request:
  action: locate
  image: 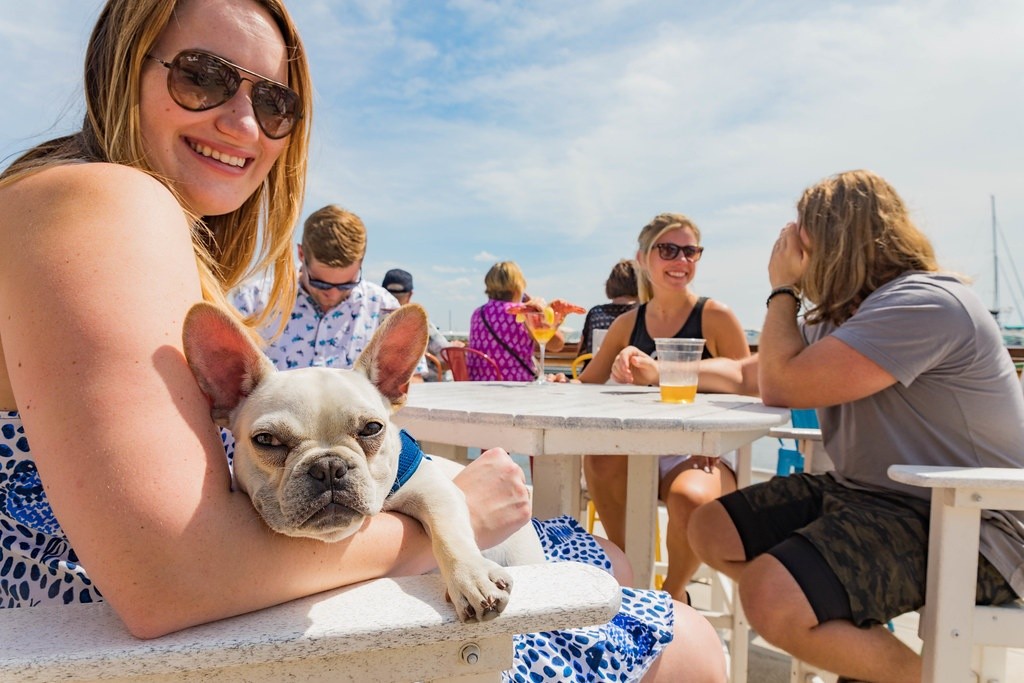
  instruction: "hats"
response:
[382,269,413,293]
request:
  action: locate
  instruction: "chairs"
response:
[0,561,622,683]
[424,350,442,381]
[440,347,534,482]
[578,327,733,647]
[572,354,663,594]
[767,422,1023,683]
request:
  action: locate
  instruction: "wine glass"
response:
[525,312,567,385]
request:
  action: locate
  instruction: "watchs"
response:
[766,286,802,316]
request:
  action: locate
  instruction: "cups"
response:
[653,337,707,404]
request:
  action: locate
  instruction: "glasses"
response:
[652,243,703,263]
[305,257,362,289]
[145,49,303,140]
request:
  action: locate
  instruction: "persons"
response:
[382,268,465,382]
[471,261,566,381]
[542,212,750,613]
[224,204,431,387]
[0,0,729,683]
[609,169,1023,683]
[578,262,642,357]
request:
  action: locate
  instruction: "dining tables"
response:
[390,380,792,683]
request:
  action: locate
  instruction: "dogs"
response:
[182,300,548,622]
